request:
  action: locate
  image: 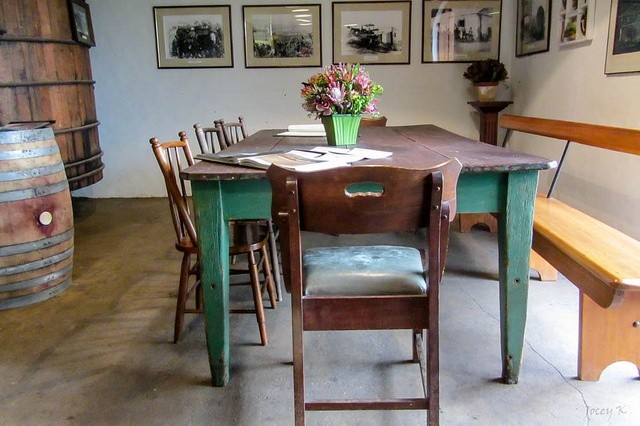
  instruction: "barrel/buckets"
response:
[0,129,74,311]
[0,1,105,190]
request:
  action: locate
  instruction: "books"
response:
[309,146,393,162]
[274,124,327,137]
[193,150,351,172]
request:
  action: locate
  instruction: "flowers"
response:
[299,61,384,119]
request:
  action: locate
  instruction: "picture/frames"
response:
[421,0,502,64]
[67,0,96,47]
[557,0,595,45]
[152,4,234,69]
[515,0,552,58]
[332,1,412,65]
[603,0,640,75]
[243,4,322,69]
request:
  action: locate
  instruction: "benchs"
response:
[458,113,640,381]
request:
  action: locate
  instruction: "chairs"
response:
[221,116,248,147]
[193,120,283,301]
[150,132,277,346]
[265,157,467,426]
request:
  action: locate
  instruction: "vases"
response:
[321,114,361,147]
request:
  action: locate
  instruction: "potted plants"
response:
[462,58,508,102]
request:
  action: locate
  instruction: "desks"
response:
[181,124,557,388]
